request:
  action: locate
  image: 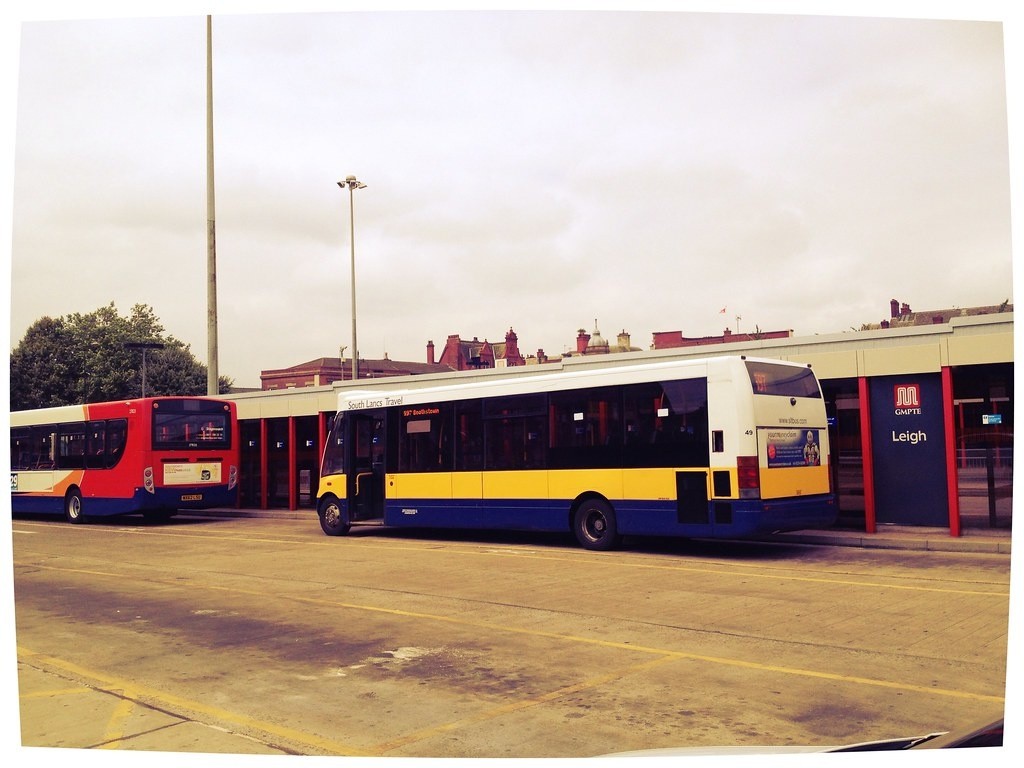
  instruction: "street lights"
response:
[336,175,368,381]
[124,342,166,399]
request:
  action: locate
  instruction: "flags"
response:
[719,308,725,313]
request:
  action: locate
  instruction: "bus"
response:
[316,354,838,551]
[10,394,241,522]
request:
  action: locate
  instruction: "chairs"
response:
[36,447,121,470]
[515,454,548,468]
[417,452,447,472]
